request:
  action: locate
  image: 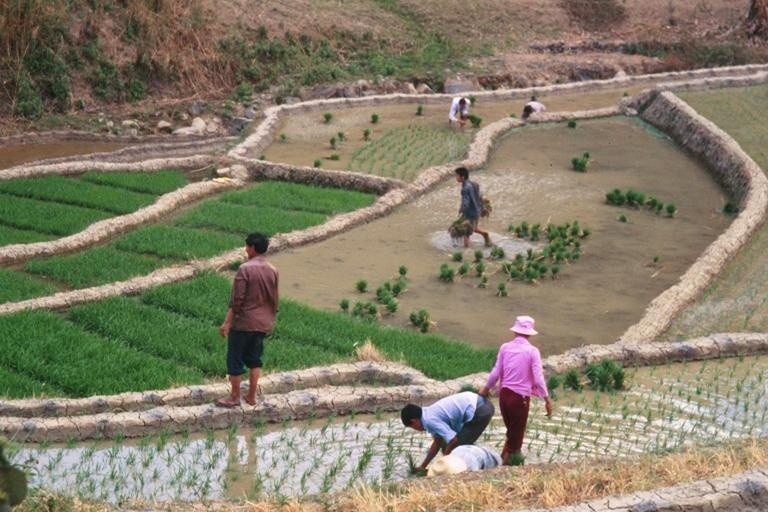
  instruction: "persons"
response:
[217,232,279,406]
[454,167,491,249]
[428,443,504,475]
[521,100,547,117]
[447,96,471,127]
[478,315,552,465]
[401,391,494,471]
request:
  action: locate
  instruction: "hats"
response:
[508,314,539,335]
